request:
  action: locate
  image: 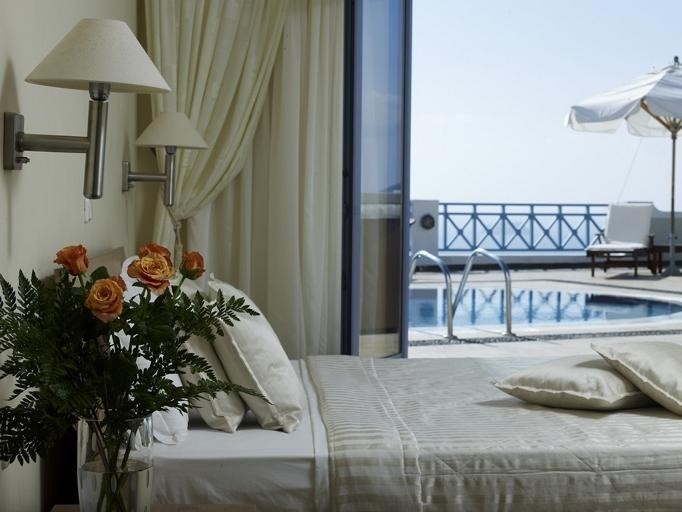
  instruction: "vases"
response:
[73,413,153,507]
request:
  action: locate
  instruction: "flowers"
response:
[1,239,266,510]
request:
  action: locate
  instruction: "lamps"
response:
[120,111,210,207]
[3,17,168,198]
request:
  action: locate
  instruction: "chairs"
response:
[584,201,662,278]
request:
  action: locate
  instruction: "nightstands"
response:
[48,502,268,511]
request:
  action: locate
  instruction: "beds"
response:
[72,352,682,511]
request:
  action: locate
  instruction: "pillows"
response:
[501,353,648,411]
[105,253,189,442]
[152,271,250,432]
[208,274,304,434]
[595,343,682,416]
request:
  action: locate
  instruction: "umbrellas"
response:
[568,54,681,280]
